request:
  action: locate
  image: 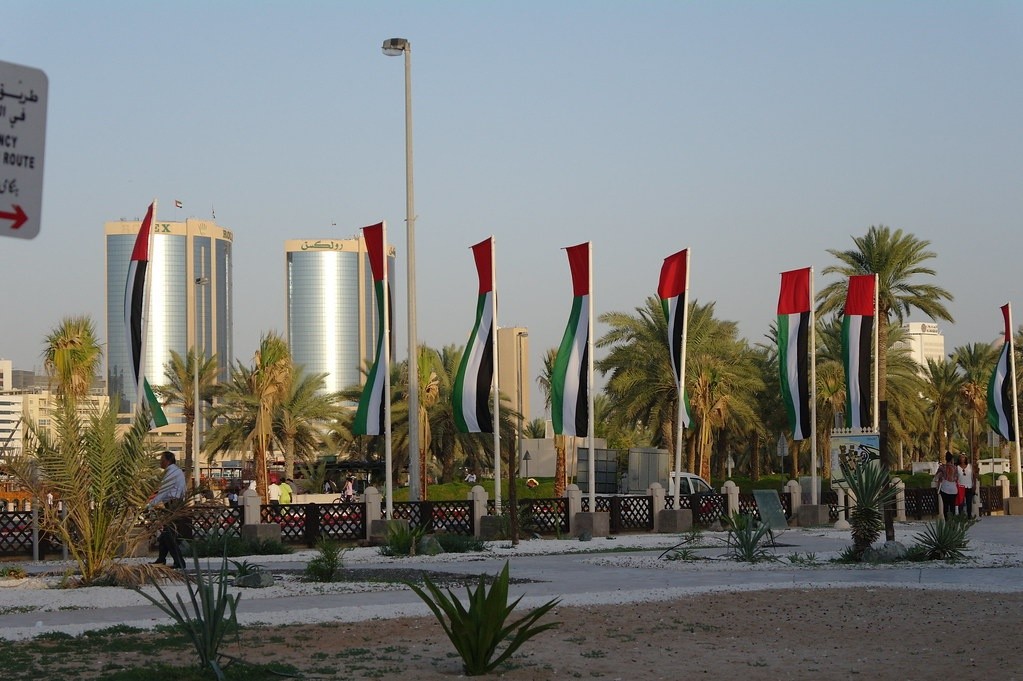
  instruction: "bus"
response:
[198,453,436,489]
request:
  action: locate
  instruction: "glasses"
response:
[962,469,967,476]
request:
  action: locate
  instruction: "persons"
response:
[322,477,355,503]
[143,451,187,569]
[228,479,293,517]
[934,451,977,521]
[464,470,476,481]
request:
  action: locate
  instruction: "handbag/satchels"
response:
[955,484,965,506]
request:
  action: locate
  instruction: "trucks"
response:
[668,472,717,512]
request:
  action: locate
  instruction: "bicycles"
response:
[332,494,362,513]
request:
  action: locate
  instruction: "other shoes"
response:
[150,559,167,564]
[171,562,186,568]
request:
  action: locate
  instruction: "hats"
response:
[271,478,277,482]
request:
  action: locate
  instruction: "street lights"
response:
[518,333,529,474]
[194,276,209,507]
[380,37,419,554]
